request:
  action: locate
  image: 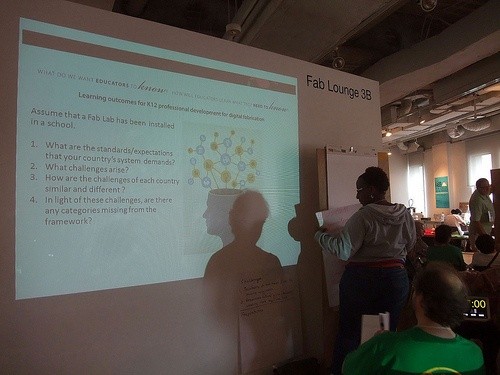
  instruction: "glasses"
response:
[356,185,369,193]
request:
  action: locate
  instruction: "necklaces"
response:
[373,199,387,205]
[417,323,452,330]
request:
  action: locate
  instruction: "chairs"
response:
[462,269,498,328]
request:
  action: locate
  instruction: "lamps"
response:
[328,47,345,70]
[226,0,241,36]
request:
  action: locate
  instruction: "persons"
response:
[313,166,500,375]
[201,188,243,250]
